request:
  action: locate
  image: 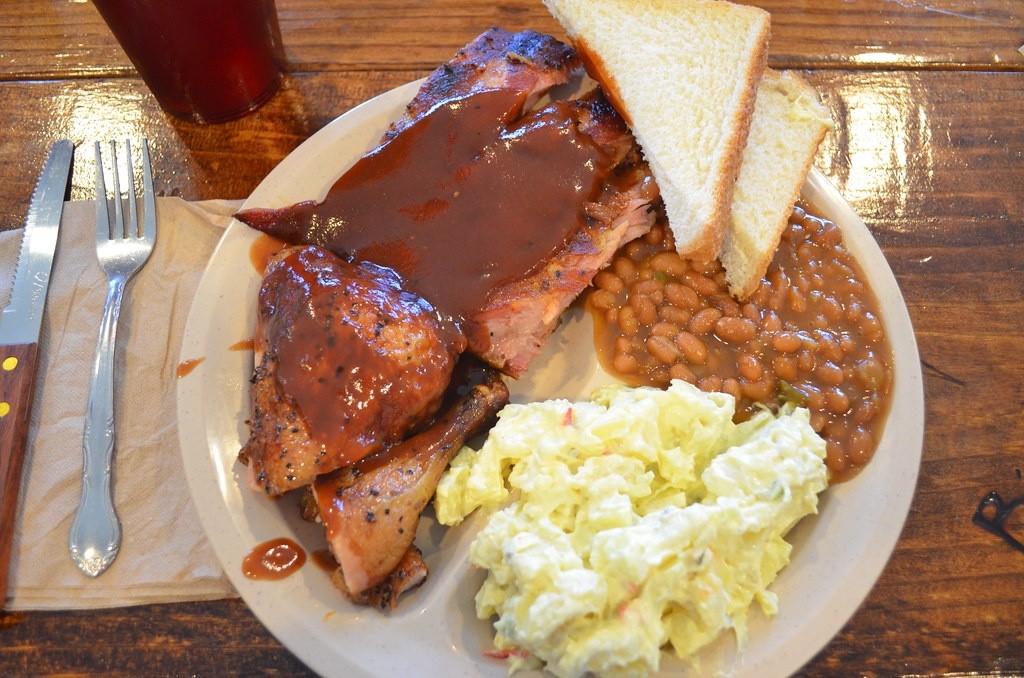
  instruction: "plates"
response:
[177,75,924,678]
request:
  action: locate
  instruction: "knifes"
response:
[0,139,75,612]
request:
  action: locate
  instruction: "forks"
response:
[66,141,158,578]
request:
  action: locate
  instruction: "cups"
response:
[92,0,288,125]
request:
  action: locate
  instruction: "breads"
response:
[542,0,772,262]
[718,66,836,302]
